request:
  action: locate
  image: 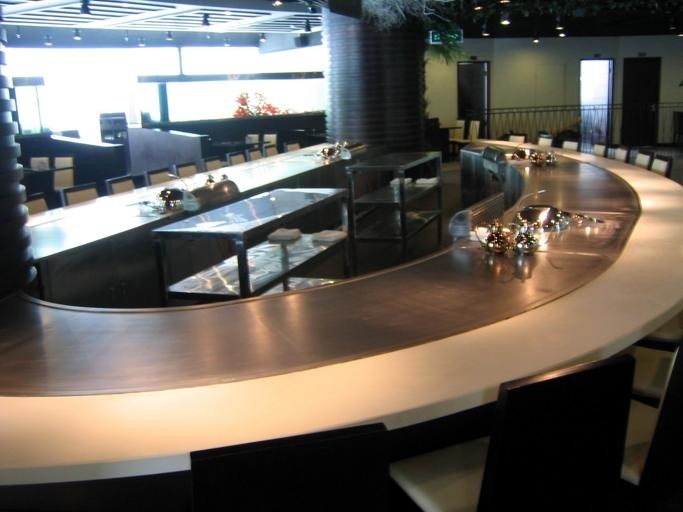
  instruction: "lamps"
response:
[13,3,569,48]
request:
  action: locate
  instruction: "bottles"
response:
[530,151,559,165]
[473,222,538,256]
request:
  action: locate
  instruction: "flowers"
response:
[231,92,289,120]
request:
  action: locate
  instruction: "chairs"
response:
[0,111,682,511]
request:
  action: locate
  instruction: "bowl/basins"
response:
[321,147,341,158]
[159,187,183,208]
